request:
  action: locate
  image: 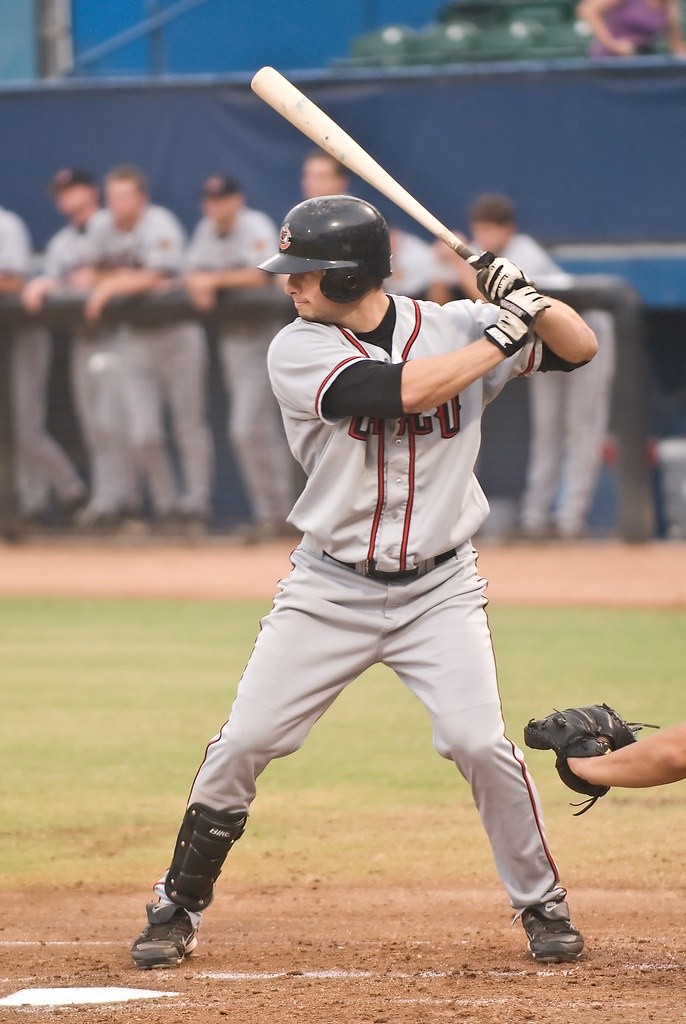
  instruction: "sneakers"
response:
[130,902,198,969]
[522,900,584,963]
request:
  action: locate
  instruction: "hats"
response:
[49,169,86,193]
[203,176,238,198]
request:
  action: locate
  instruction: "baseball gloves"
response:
[523,702,661,817]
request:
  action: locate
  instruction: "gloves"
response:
[474,250,527,306]
[484,279,552,358]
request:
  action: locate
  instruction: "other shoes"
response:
[0,490,302,545]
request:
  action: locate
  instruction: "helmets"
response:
[257,195,393,304]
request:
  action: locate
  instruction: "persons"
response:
[576,0,686,60]
[523,703,685,816]
[0,148,617,545]
[130,196,598,968]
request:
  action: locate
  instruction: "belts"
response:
[323,548,456,581]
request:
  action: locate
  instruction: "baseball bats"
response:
[251,65,481,271]
